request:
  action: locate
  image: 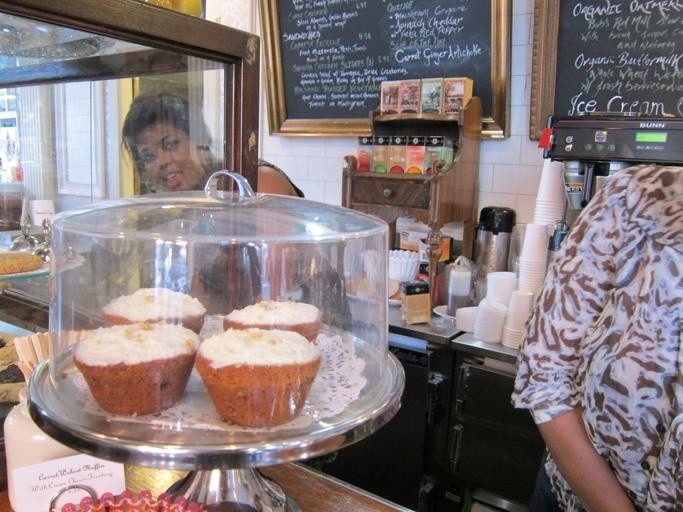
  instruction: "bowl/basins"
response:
[361,250,420,282]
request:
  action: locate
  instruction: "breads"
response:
[0,252,43,274]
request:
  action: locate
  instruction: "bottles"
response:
[447,256,471,316]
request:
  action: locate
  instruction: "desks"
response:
[1,316,417,512]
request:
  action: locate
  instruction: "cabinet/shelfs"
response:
[299,326,451,512]
[450,339,547,512]
[341,97,483,316]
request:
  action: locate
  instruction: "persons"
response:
[116,87,354,473]
[510,161,682,512]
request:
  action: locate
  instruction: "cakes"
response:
[195,325,321,429]
[72,320,201,417]
[102,287,208,335]
[222,297,322,343]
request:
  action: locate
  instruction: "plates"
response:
[433,305,455,321]
[352,277,400,300]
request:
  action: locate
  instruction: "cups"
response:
[475,153,567,350]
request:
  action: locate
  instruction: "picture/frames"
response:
[55,79,107,203]
